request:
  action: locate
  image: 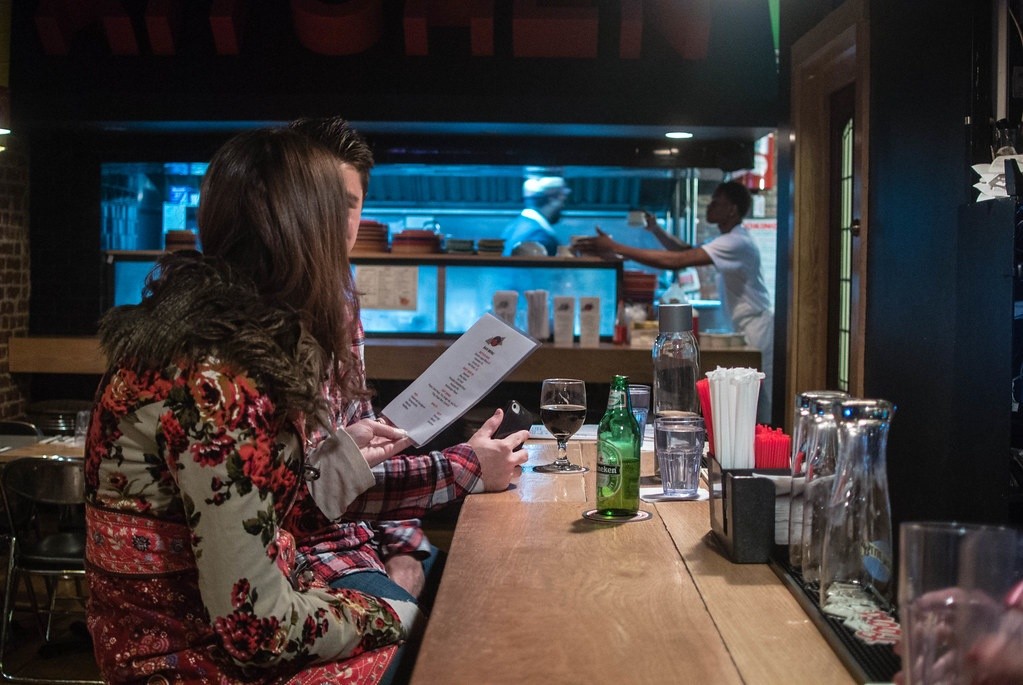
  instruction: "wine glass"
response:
[533,378,590,474]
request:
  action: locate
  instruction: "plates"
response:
[349,221,387,255]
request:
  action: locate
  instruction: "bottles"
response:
[653,305,699,480]
[597,375,640,517]
[612,278,721,348]
[790,390,893,619]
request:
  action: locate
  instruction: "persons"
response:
[571,180,775,419]
[500,177,573,260]
[85,114,532,685]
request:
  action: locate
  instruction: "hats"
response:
[522,177,572,197]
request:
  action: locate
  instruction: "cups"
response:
[556,235,619,258]
[654,417,704,497]
[899,522,1016,685]
[627,211,649,227]
[629,385,651,448]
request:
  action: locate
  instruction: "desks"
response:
[409,433,859,685]
[0,438,90,472]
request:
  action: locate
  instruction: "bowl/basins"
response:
[165,230,196,251]
[477,240,503,255]
[448,240,473,254]
[391,230,439,254]
[512,240,548,256]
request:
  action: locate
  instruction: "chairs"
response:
[0,418,105,685]
